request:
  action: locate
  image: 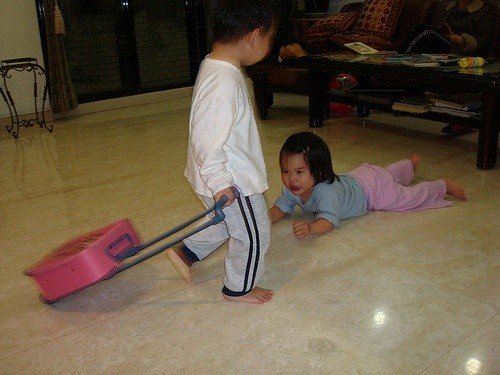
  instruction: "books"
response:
[344,42,448,68]
[392,87,484,118]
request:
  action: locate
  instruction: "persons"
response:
[166,0,275,304]
[268,131,467,239]
[397,0,495,55]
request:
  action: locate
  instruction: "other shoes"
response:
[441,122,477,135]
[371,73,410,86]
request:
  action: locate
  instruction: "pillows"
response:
[303,11,358,40]
[328,30,393,52]
[358,0,403,40]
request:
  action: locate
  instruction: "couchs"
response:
[247,12,310,121]
[335,0,500,118]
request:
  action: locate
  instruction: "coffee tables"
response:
[296,52,500,170]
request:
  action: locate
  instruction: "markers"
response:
[431,56,452,58]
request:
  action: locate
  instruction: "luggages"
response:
[24,187,239,305]
